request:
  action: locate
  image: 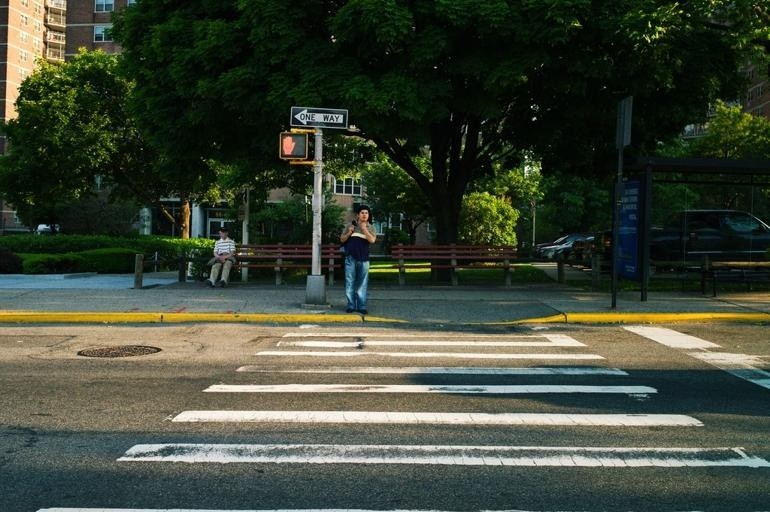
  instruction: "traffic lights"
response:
[278,127,310,157]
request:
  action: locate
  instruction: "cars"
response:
[648,207,769,274]
[533,230,615,263]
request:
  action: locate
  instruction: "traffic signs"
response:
[290,105,349,130]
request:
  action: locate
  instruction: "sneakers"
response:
[346,308,367,314]
[207,279,226,288]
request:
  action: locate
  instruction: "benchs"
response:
[391,242,519,287]
[228,242,342,287]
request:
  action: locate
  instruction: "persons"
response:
[207,227,237,287]
[340,205,377,314]
